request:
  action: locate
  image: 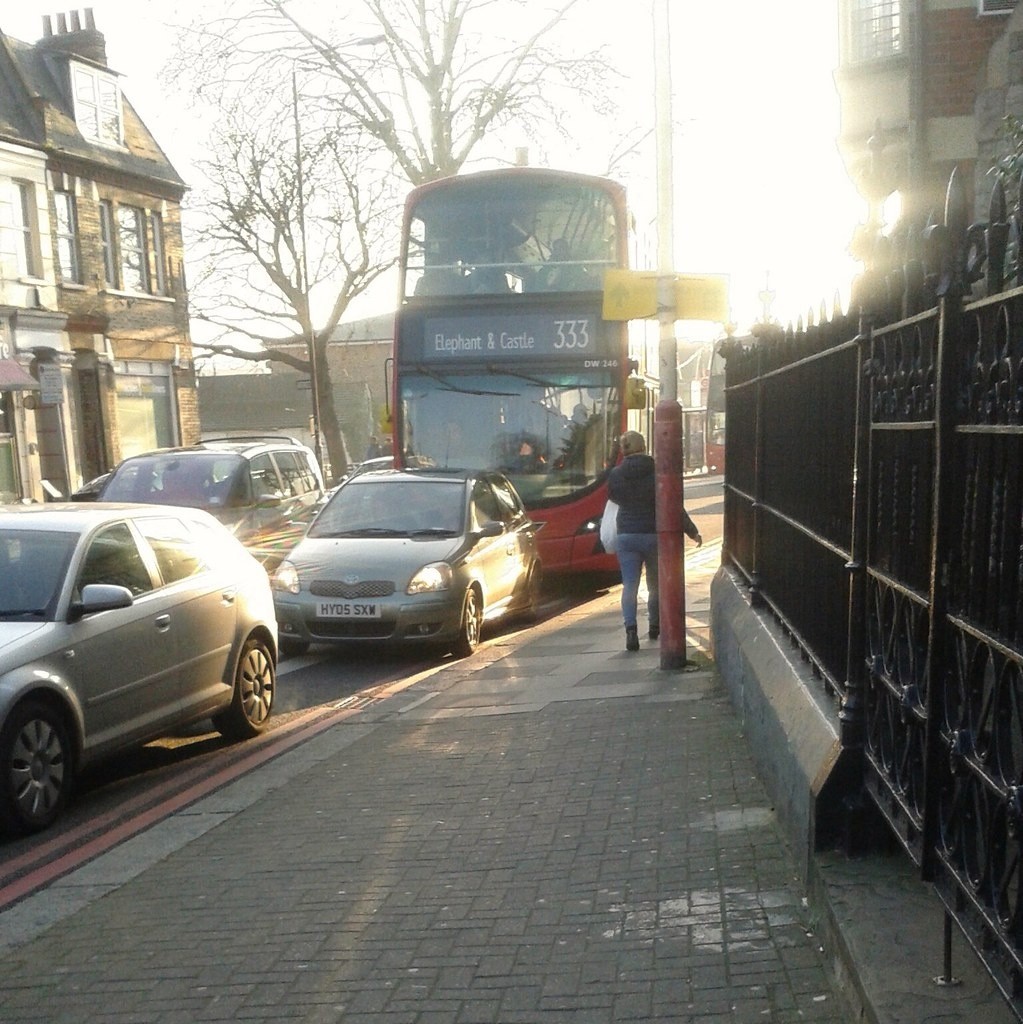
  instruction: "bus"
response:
[704,335,751,476]
[378,166,649,582]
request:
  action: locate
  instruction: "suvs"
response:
[97,436,325,561]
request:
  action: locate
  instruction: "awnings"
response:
[0,358,41,390]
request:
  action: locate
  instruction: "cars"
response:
[0,503,276,835]
[268,468,544,661]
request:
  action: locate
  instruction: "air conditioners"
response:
[977,0,1020,19]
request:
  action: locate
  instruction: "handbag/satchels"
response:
[600,499,620,554]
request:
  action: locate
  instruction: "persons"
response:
[571,402,590,422]
[539,239,599,291]
[365,436,393,460]
[607,430,702,652]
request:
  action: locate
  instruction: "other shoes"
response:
[649,625,659,639]
[627,630,639,651]
